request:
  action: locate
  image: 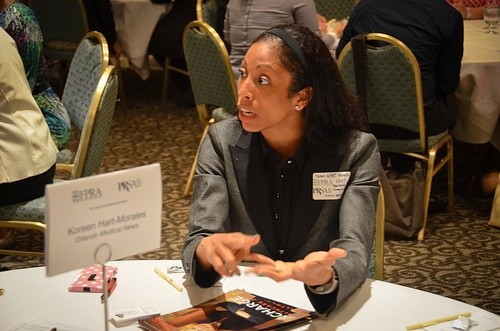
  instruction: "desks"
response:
[0,260,500,331]
[110,0,173,68]
[330,17,500,150]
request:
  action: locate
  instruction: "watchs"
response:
[308,270,334,293]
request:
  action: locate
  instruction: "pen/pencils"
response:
[155,267,183,292]
[408,313,474,330]
[101,277,116,302]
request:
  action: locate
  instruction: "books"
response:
[137,289,319,331]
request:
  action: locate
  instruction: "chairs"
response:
[0,0,453,279]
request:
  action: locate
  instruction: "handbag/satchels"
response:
[379,160,426,239]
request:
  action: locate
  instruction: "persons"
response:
[0,0,73,151]
[181,23,381,318]
[336,0,464,215]
[149,0,208,91]
[0,26,59,207]
[223,0,349,80]
